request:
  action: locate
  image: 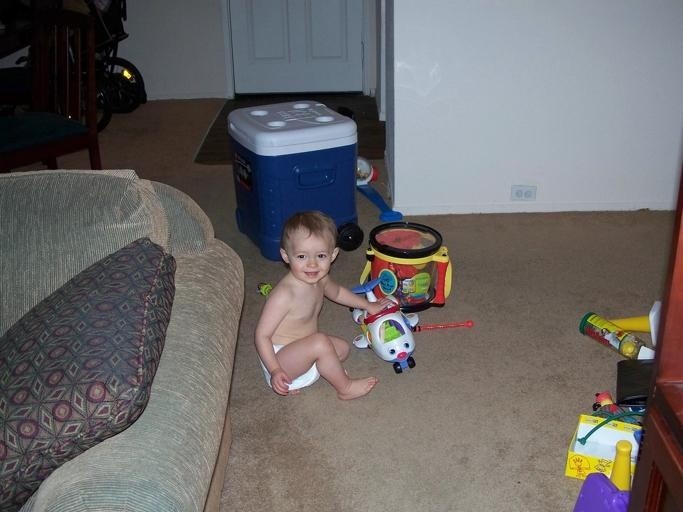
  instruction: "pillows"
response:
[0,237,176,512]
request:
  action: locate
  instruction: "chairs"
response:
[1,0,102,178]
[0,163,246,511]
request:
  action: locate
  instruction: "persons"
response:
[253,210,392,402]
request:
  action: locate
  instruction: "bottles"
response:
[578,311,647,361]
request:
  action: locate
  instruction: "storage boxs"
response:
[224,98,364,262]
[563,413,643,493]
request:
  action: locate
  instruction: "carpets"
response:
[192,88,385,166]
